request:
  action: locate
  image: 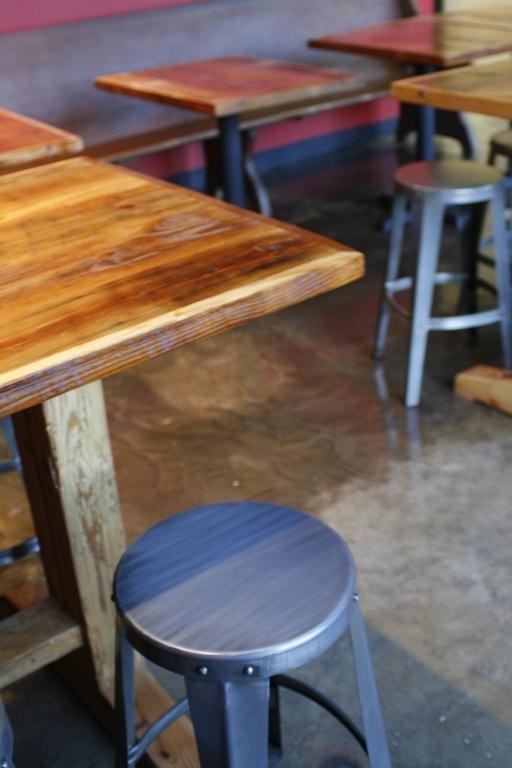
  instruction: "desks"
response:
[96,53,369,227]
[308,7,512,167]
[0,155,367,766]
[0,107,81,181]
[390,55,512,416]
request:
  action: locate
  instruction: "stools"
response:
[367,159,512,410]
[459,128,511,321]
[107,497,394,767]
